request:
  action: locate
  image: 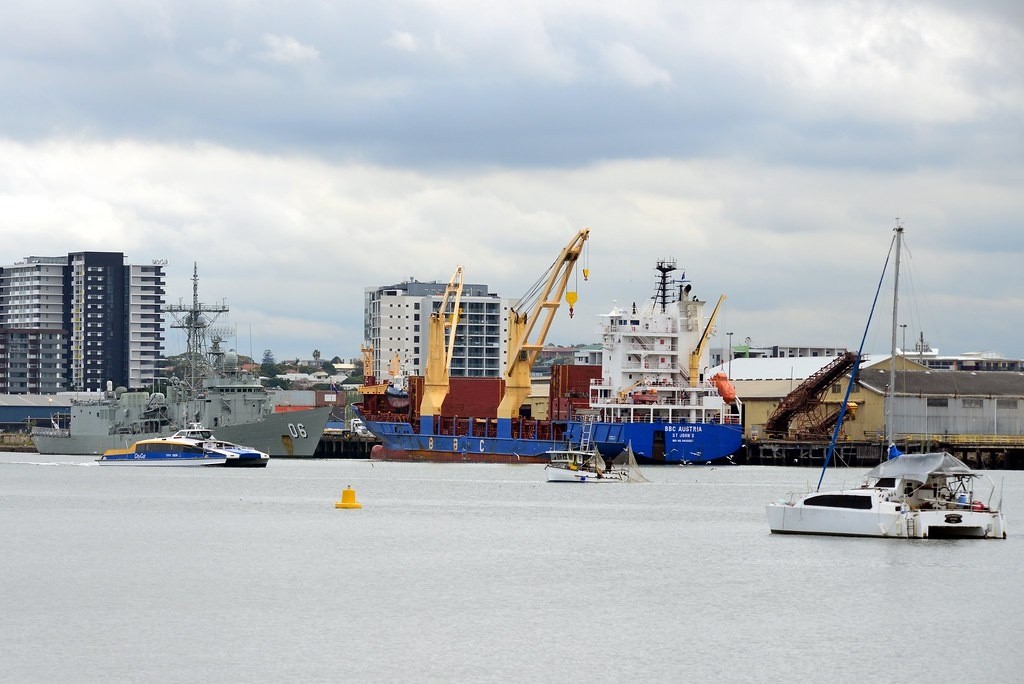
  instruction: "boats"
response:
[95,423,270,468]
[545,415,652,484]
[350,228,743,463]
[30,262,334,457]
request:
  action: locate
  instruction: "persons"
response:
[604,457,616,474]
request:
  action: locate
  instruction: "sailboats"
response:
[765,217,1007,538]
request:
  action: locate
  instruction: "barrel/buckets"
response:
[959,494,967,503]
